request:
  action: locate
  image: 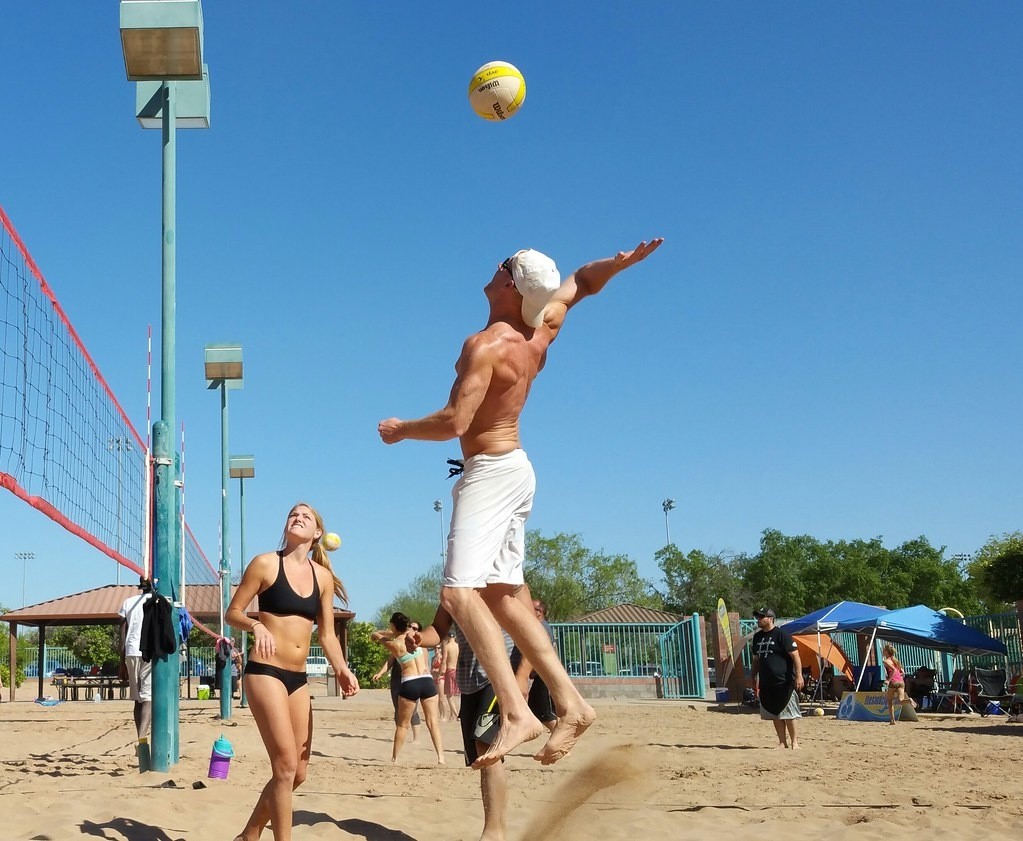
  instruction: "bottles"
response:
[137,737,150,774]
[95,693,100,703]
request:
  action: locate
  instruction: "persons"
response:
[905,666,933,696]
[230,637,243,700]
[401,573,533,841]
[375,237,664,773]
[749,608,805,750]
[370,611,451,766]
[225,501,361,841]
[944,669,974,712]
[117,574,152,755]
[433,632,462,720]
[882,644,917,725]
[530,598,561,733]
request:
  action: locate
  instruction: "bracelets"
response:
[251,622,262,630]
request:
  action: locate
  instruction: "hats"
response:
[138,576,150,589]
[513,248,561,329]
[752,607,776,621]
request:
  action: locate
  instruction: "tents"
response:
[834,605,1011,695]
[779,601,890,680]
[723,622,857,691]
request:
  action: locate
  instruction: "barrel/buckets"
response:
[989,700,1000,714]
[715,687,730,702]
[208,733,235,779]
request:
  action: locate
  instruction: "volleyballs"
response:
[322,533,341,553]
[813,707,825,716]
[468,60,527,121]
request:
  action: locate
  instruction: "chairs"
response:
[799,664,1017,718]
[200,675,216,698]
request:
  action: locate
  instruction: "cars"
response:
[305,656,329,677]
[23,659,62,678]
[188,655,209,676]
[562,654,716,689]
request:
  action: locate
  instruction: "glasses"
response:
[408,625,418,630]
[502,258,519,293]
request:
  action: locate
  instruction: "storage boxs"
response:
[196,685,211,699]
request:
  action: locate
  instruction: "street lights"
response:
[229,454,255,709]
[662,498,678,612]
[203,343,245,719]
[433,499,445,579]
[119,0,212,780]
